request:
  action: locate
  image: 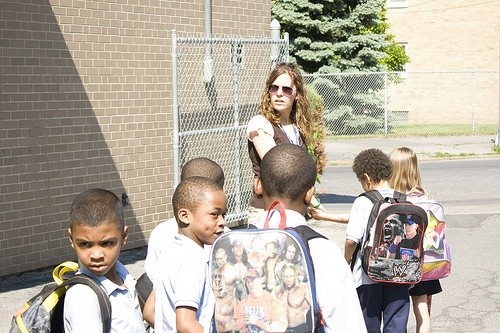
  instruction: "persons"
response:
[229,269,288,333]
[243,143,367,333]
[211,248,247,301]
[343,148,413,333]
[256,238,283,292]
[145,177,228,333]
[387,214,420,260]
[145,157,231,276]
[271,263,312,333]
[309,147,442,333]
[52,187,148,333]
[382,213,405,243]
[275,241,308,287]
[229,238,257,299]
[248,62,325,241]
[212,274,240,333]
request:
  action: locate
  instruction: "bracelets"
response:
[311,197,321,208]
[388,242,398,253]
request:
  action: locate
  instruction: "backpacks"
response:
[9,260,112,333]
[358,190,428,285]
[209,200,328,333]
[405,191,452,281]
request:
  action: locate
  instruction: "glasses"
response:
[269,85,297,96]
